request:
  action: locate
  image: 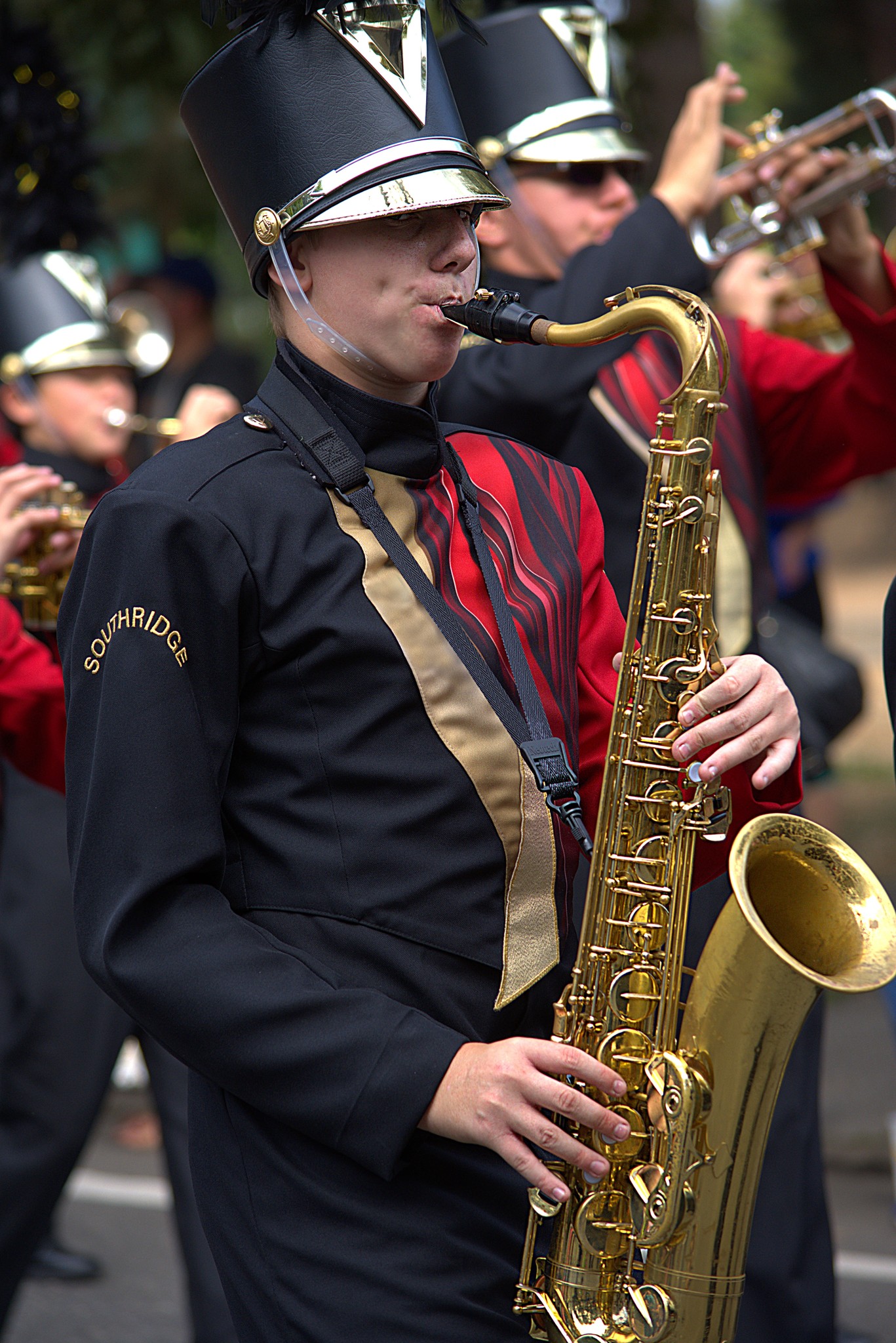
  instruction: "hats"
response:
[179,0,512,298]
[438,4,648,166]
[1,248,136,376]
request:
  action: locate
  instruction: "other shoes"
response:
[27,1236,104,1281]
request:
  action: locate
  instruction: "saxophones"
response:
[435,276,895,1343]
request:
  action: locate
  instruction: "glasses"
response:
[509,159,640,188]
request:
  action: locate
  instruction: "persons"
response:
[55,0,803,1343]
[0,0,895,1343]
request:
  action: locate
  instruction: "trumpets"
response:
[687,67,895,269]
